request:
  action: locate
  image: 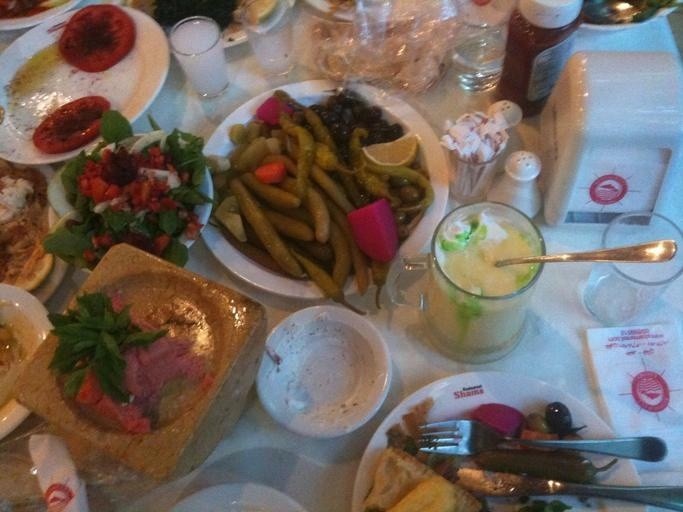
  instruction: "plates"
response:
[581,0,679,36]
[3,3,172,163]
[0,159,73,302]
[1,282,56,439]
[263,305,393,437]
[350,371,647,510]
[194,78,450,300]
[164,484,304,512]
[45,133,214,250]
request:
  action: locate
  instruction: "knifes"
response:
[456,467,681,511]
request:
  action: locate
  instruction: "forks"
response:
[416,420,666,462]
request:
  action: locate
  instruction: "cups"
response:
[585,209,683,329]
[168,16,228,98]
[239,2,449,83]
[385,201,545,366]
[448,149,497,206]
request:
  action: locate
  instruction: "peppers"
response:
[231,107,434,301]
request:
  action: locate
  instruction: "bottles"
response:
[482,150,544,220]
[486,100,527,167]
[500,1,585,118]
[450,1,506,96]
